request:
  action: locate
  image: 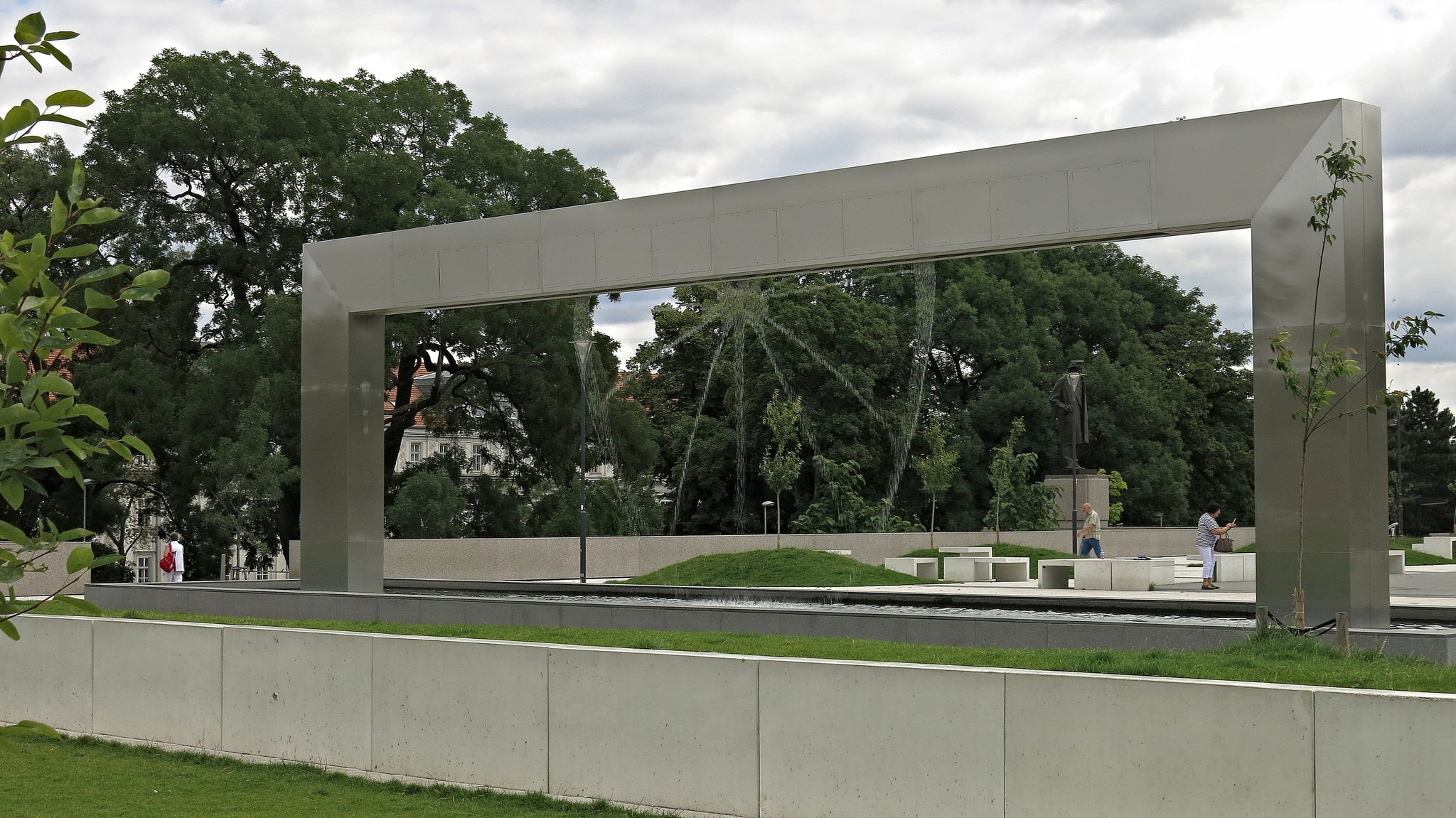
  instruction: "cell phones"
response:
[1233,518,1236,523]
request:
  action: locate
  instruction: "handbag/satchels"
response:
[1213,531,1235,553]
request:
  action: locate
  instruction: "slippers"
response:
[1133,555,1151,560]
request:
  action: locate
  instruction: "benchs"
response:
[888,554,1256,593]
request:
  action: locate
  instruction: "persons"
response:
[1194,502,1236,590]
[1076,502,1103,558]
[162,532,185,583]
[1049,360,1091,469]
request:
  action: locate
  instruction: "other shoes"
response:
[1211,584,1220,589]
[1202,584,1215,589]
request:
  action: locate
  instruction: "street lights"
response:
[82,478,95,538]
[568,338,596,586]
[1395,392,1404,539]
[761,501,775,534]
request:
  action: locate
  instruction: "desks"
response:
[939,546,994,583]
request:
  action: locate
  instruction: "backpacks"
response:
[160,543,176,574]
